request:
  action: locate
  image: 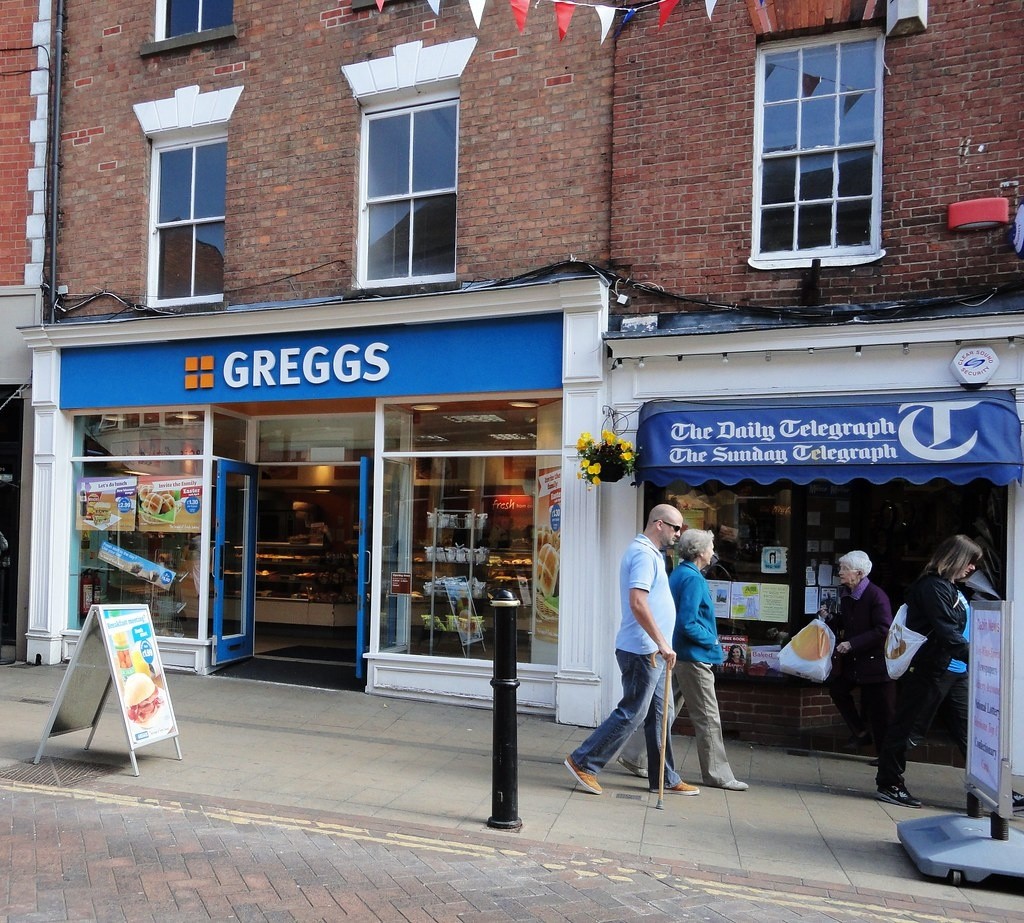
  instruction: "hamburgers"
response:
[124,673,163,728]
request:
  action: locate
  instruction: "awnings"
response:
[631,387,1024,489]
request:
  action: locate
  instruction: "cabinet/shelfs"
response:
[424,507,489,659]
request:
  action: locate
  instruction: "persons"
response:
[616,527,748,791]
[722,645,745,666]
[814,551,893,767]
[563,503,701,795]
[875,532,984,808]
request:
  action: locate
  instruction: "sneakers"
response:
[1011,793,1023,812]
[876,783,922,808]
[650,779,699,796]
[564,756,602,794]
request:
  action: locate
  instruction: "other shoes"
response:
[718,777,748,791]
[840,732,873,751]
[617,755,648,778]
[870,756,881,767]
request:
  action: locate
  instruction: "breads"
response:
[139,484,175,515]
[536,524,561,600]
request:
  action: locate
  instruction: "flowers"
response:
[577,430,641,493]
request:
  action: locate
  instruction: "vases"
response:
[598,462,625,482]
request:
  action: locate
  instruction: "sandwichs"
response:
[93,502,110,525]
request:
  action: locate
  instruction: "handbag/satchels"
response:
[885,604,927,680]
[776,614,836,684]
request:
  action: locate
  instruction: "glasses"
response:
[836,567,859,571]
[653,520,681,528]
